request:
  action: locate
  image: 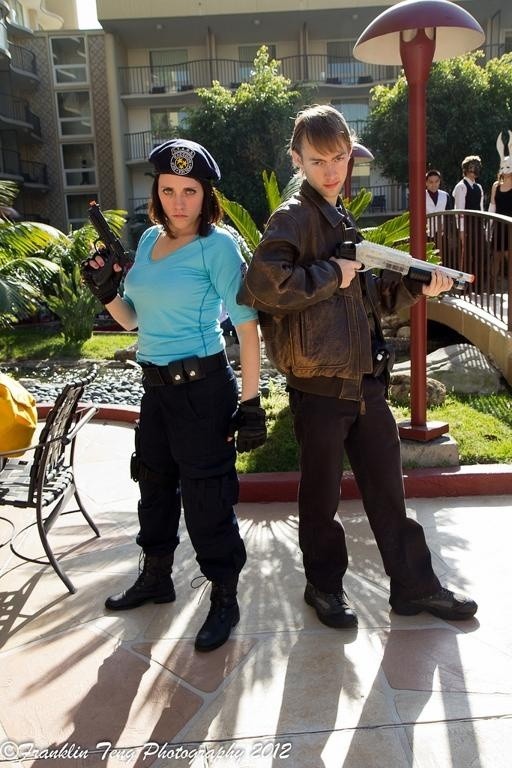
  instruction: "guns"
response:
[336,240,475,293]
[88,201,133,286]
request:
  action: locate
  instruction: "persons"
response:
[238,103,479,630]
[485,155,511,282]
[84,136,268,650]
[426,166,456,250]
[451,156,489,294]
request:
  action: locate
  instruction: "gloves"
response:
[79,250,123,306]
[228,397,267,453]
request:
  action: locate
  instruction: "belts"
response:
[142,352,229,387]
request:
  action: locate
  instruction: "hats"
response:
[148,138,221,182]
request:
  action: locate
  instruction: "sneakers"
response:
[304,581,358,628]
[390,587,477,620]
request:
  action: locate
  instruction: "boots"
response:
[191,572,240,652]
[106,551,175,610]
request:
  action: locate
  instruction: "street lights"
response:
[352,0,487,444]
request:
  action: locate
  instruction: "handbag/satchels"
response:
[0,373,38,458]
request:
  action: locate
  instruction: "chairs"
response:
[0,361,104,599]
